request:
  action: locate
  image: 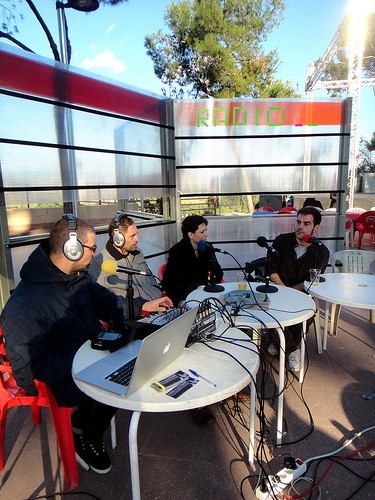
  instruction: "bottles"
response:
[288,197,293,207]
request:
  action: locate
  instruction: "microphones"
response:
[197,240,229,254]
[304,234,323,246]
[116,265,152,276]
[257,236,278,255]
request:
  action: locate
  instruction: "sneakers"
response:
[71,427,112,475]
[288,348,301,371]
[268,342,278,355]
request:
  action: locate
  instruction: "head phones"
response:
[112,212,127,247]
[62,214,84,261]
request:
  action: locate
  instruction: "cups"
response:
[237,272,249,290]
[309,269,321,288]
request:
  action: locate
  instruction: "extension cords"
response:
[255,458,307,500]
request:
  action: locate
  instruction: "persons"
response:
[89,191,337,400]
[1,215,119,475]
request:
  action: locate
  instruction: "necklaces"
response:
[194,248,198,251]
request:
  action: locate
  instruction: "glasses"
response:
[82,245,97,253]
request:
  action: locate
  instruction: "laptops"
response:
[74,304,199,398]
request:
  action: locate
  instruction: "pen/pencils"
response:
[188,369,217,387]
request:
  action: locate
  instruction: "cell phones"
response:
[97,332,121,340]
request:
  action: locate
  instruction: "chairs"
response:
[158,206,375,337]
[0,324,80,492]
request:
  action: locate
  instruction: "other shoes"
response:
[226,385,250,403]
[192,407,216,426]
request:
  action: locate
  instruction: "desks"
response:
[71,318,259,500]
[304,272,374,352]
[185,282,316,448]
